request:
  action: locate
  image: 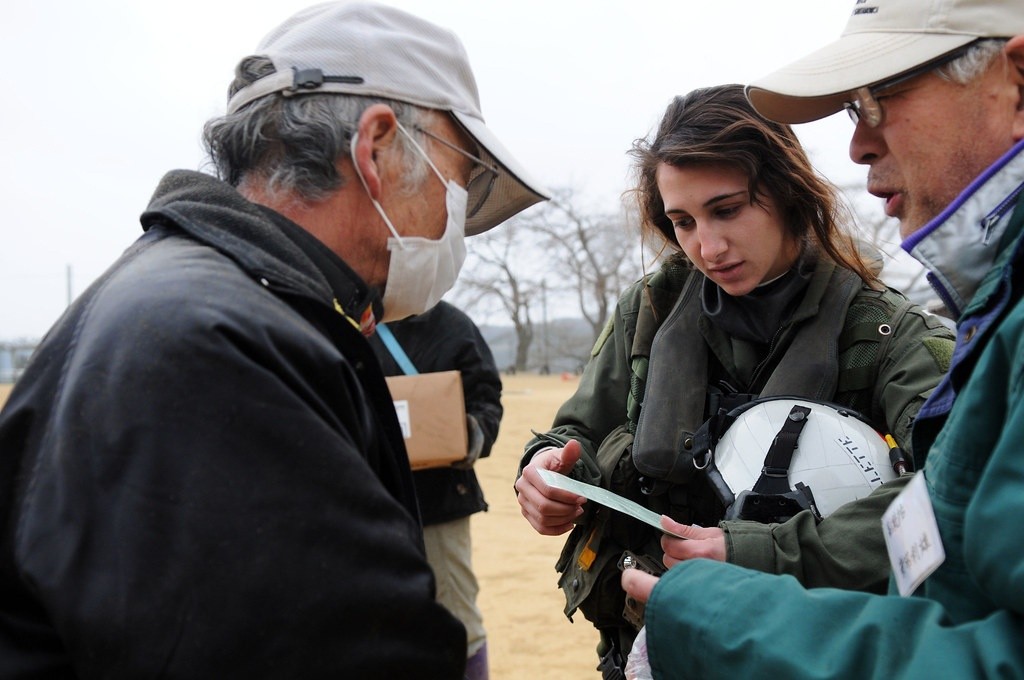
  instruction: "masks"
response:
[350,120,468,323]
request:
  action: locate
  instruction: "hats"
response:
[744,1,1024,126]
[225,1,551,236]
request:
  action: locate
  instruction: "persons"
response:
[366,301,503,680]
[514,83,956,680]
[621,0,1024,680]
[0,0,551,680]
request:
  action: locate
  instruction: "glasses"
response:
[396,117,500,217]
[843,46,982,129]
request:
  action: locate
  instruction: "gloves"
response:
[451,413,484,471]
